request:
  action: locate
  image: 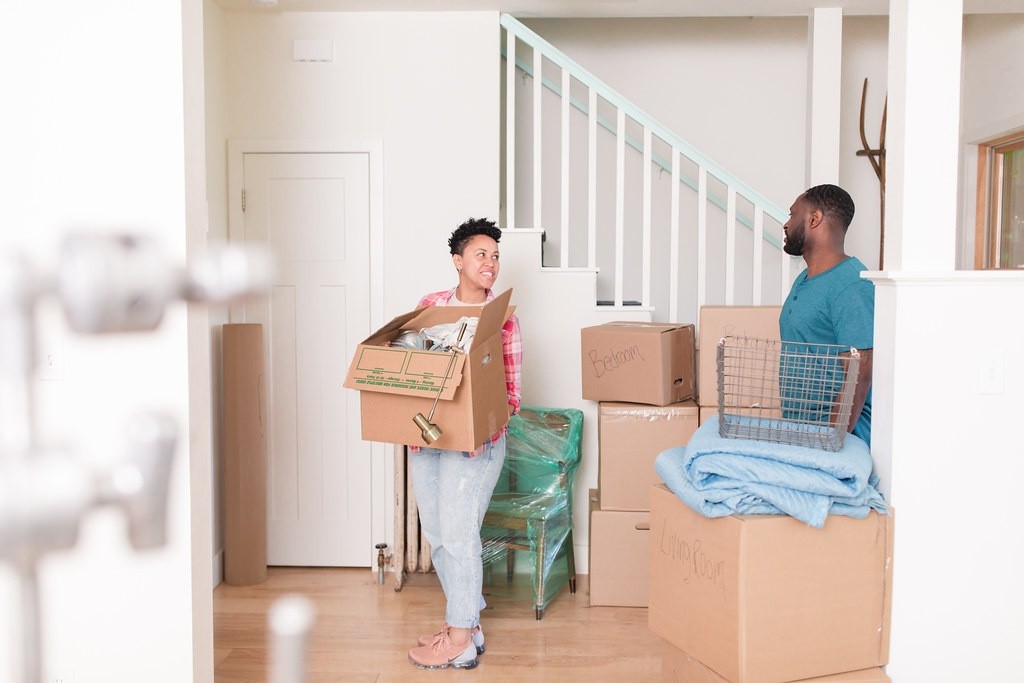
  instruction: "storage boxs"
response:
[587,488,653,610]
[596,398,699,514]
[342,287,517,452]
[646,482,896,683]
[698,302,791,429]
[581,319,699,409]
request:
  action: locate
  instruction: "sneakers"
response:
[409,630,478,671]
[418,623,485,656]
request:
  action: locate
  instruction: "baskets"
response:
[716,335,860,452]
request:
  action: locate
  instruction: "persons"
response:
[409,217,522,670]
[779,184,874,449]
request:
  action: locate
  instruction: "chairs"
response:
[477,407,584,622]
